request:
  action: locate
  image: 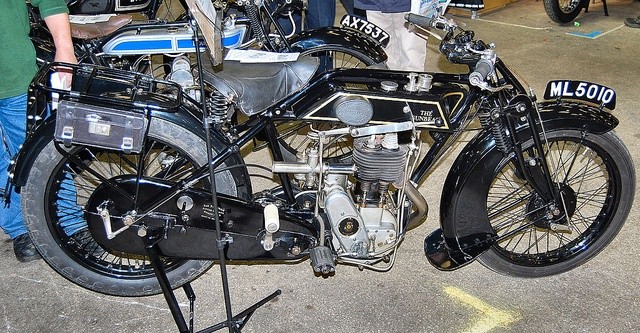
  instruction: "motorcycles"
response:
[0,7,635,332]
[30,0,389,163]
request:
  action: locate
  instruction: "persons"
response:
[268,1,337,73]
[0,0,105,263]
[352,1,429,72]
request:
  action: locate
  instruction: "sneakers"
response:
[625,16,640,28]
[67,227,106,256]
[13,232,43,263]
